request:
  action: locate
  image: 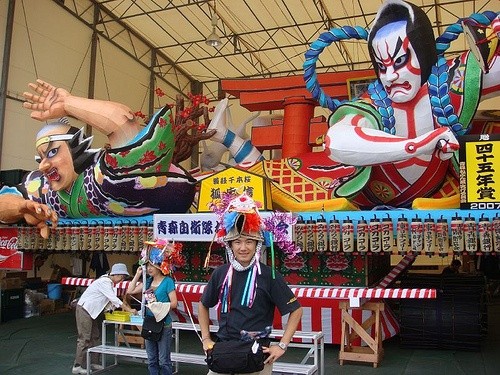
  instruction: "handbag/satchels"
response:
[205,339,264,374]
[141,316,165,341]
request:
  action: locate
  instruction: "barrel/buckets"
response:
[46,284,62,299]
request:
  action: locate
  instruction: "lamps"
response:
[205,0,222,47]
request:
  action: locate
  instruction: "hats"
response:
[204,191,301,272]
[139,237,187,275]
[110,263,129,276]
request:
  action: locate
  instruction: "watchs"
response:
[278,341,287,350]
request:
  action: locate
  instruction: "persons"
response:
[72,263,138,375]
[0,77,283,239]
[198,194,302,375]
[442,260,461,274]
[127,238,183,375]
[303,0,500,210]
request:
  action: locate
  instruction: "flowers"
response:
[134,88,215,131]
[148,238,186,275]
[206,191,301,259]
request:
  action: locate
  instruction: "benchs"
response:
[87,341,321,375]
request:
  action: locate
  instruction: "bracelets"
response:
[202,337,211,342]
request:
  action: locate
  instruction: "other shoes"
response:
[72,365,92,374]
[92,364,103,369]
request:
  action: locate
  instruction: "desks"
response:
[102,319,325,375]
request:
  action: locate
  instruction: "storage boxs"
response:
[2,278,21,288]
